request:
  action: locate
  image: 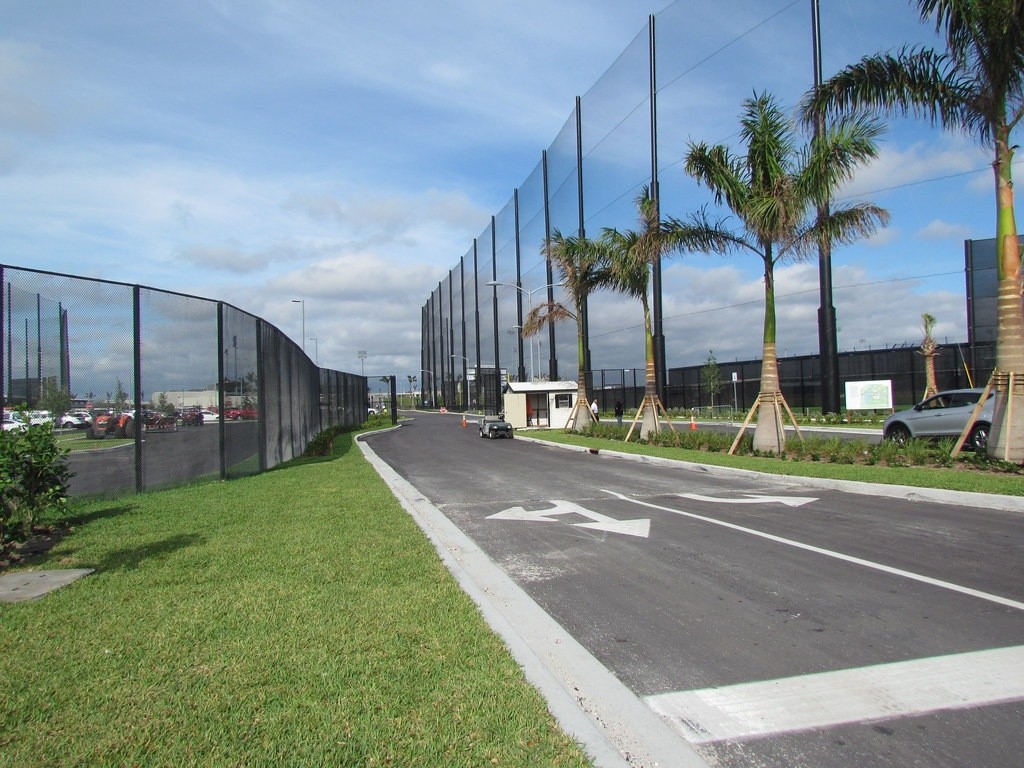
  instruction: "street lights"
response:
[292,299,305,352]
[450,355,471,414]
[358,350,367,376]
[310,338,318,368]
[486,275,570,382]
[419,369,436,412]
[513,323,542,383]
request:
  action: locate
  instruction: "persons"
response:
[590,398,599,424]
[614,401,624,427]
[376,403,388,414]
[197,409,202,416]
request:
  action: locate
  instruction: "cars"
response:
[478,416,514,440]
[368,407,380,415]
[0,406,219,440]
[882,388,996,452]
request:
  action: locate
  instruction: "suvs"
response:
[226,402,259,421]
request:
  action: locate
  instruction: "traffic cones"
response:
[689,414,699,431]
[462,414,467,428]
